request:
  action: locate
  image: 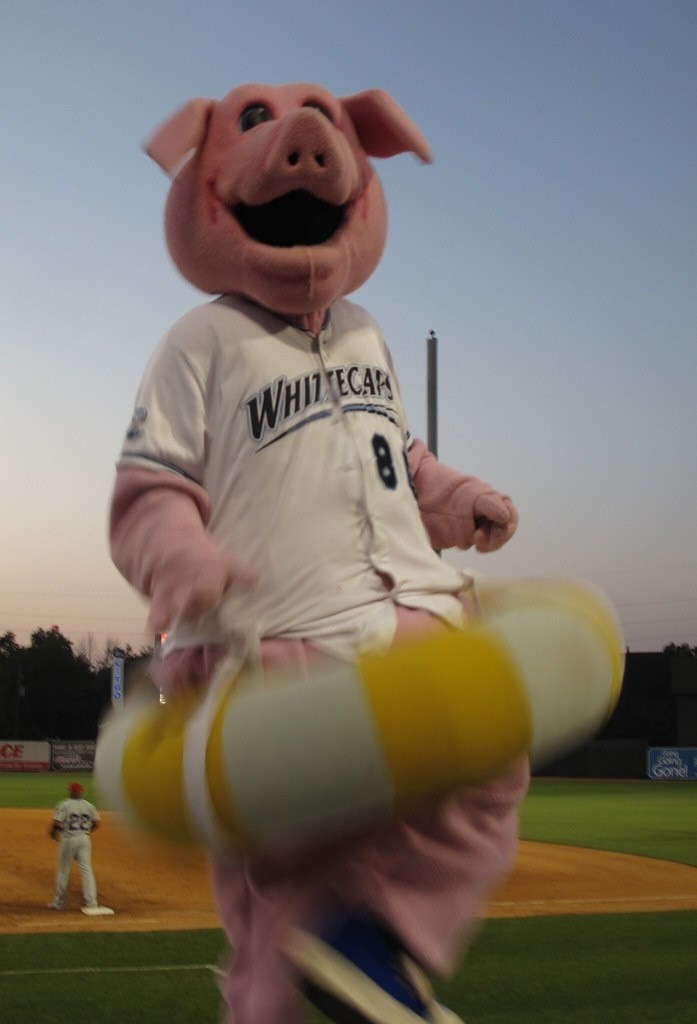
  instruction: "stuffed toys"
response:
[108,84,530,1024]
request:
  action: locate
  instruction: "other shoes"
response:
[46,902,64,910]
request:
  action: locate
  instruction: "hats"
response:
[66,783,84,794]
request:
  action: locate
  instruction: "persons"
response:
[47,784,102,910]
